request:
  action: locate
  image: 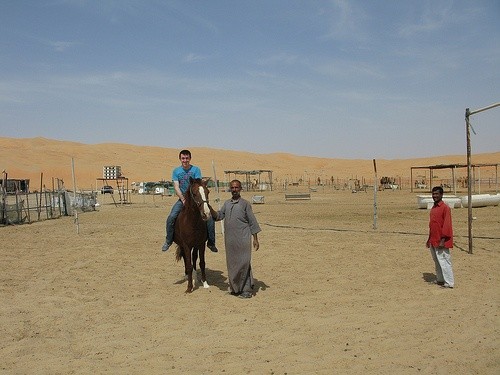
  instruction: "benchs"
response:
[285,193,311,200]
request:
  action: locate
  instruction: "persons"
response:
[207,178,262,297]
[161,148,218,253]
[424,186,455,288]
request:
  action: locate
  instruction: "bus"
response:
[155,183,176,196]
[139,182,155,194]
[379,176,399,190]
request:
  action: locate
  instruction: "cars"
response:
[351,189,357,193]
[101,185,114,195]
[430,175,441,181]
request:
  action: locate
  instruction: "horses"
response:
[174,177,213,293]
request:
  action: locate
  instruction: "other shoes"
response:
[207,242,218,252]
[162,241,172,251]
